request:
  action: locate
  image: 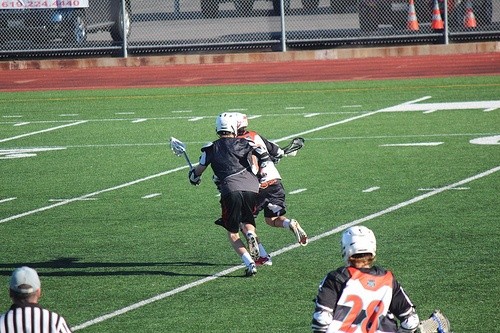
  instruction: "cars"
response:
[0,0,132,49]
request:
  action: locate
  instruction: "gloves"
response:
[269,154,280,165]
[188,169,201,186]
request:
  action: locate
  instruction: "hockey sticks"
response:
[271,137,305,162]
[416,309,451,333]
[170,136,201,186]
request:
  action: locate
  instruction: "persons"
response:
[0,266,72,333]
[313,225,420,333]
[188,112,308,278]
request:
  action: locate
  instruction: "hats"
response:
[9,267,41,293]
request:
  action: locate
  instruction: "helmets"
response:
[216,112,239,137]
[234,112,248,131]
[341,226,376,263]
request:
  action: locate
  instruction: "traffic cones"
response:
[431,0,447,33]
[405,0,421,34]
[461,2,480,32]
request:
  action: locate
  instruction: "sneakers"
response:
[290,219,309,247]
[245,231,260,261]
[254,254,272,266]
[245,261,257,278]
